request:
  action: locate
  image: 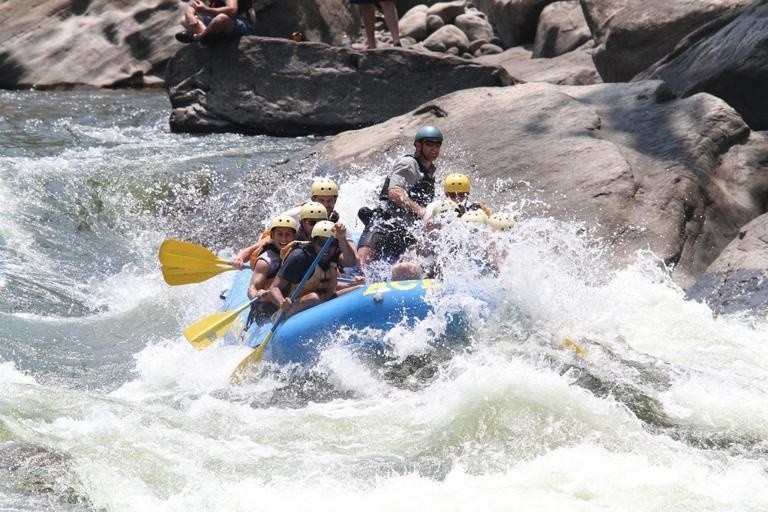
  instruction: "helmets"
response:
[270,215,297,234]
[414,125,443,141]
[300,201,328,220]
[432,199,514,232]
[311,220,336,239]
[444,173,470,195]
[311,177,338,199]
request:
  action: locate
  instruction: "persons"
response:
[260,177,357,256]
[232,202,328,271]
[247,214,299,328]
[267,220,357,314]
[174,1,256,45]
[354,0,402,48]
[391,165,517,281]
[357,126,443,266]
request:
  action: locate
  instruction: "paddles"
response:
[160,266,249,285]
[231,231,336,385]
[160,239,251,270]
[184,291,270,350]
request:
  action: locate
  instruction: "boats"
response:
[220,230,476,374]
